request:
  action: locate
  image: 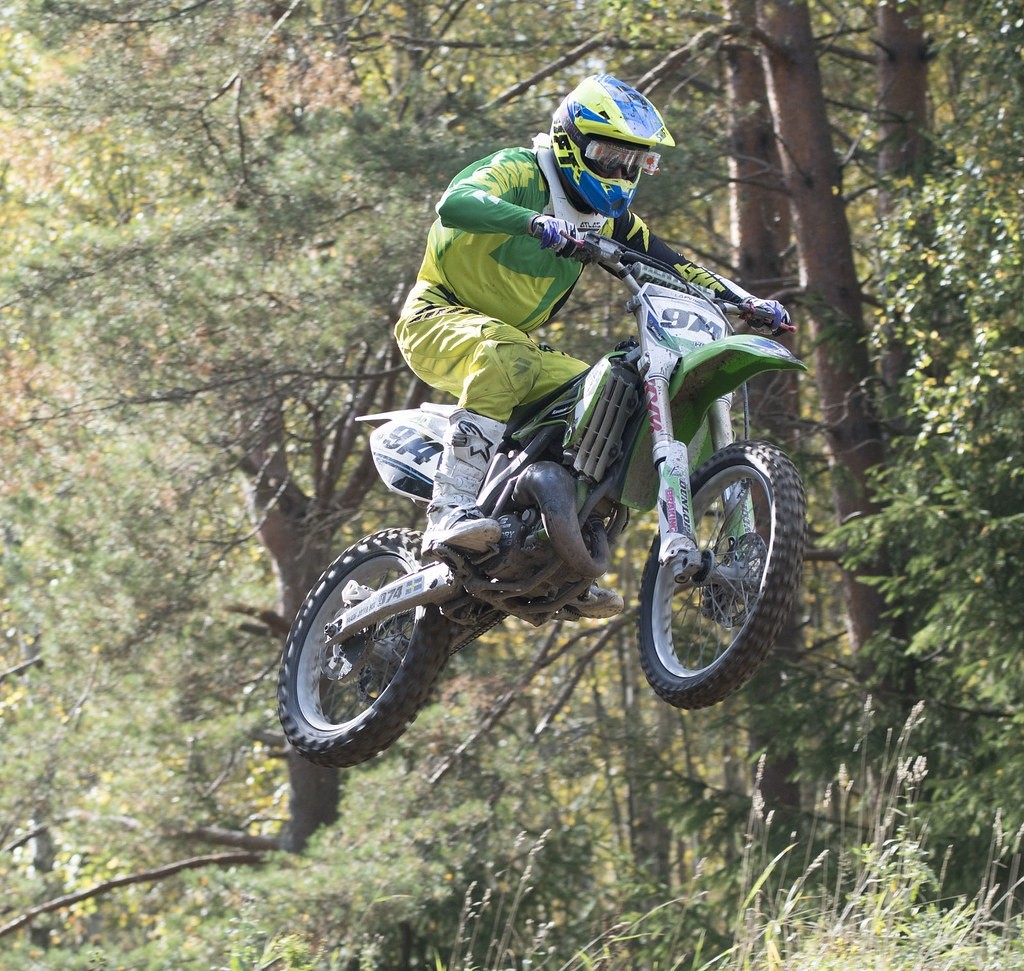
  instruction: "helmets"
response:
[550,74,676,218]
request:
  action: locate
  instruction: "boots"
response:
[420,411,507,560]
[567,581,624,619]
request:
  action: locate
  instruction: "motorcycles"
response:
[276,221,809,770]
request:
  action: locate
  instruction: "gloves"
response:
[743,297,791,333]
[532,215,581,259]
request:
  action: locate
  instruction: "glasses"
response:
[584,139,661,177]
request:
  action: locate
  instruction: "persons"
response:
[394,75,792,617]
